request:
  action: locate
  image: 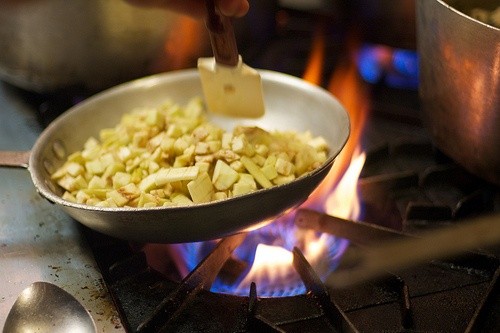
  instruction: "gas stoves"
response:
[30,0,500,333]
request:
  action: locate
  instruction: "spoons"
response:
[3,281,98,333]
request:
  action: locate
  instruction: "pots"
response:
[0,0,215,93]
[0,68,350,245]
[414,0,500,184]
